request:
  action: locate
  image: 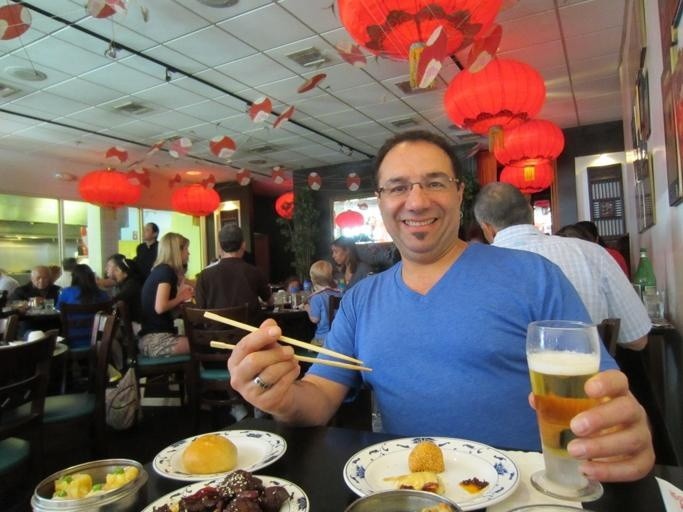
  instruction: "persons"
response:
[139,226,277,422]
[463,183,650,353]
[1,246,142,389]
[132,223,160,283]
[228,130,658,483]
[290,237,401,355]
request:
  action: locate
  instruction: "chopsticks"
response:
[202,307,373,373]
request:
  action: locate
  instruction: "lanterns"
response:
[77,170,142,219]
[443,58,546,153]
[501,164,556,200]
[494,118,566,180]
[337,0,504,60]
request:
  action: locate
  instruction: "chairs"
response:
[112,301,190,429]
[1,329,60,512]
[596,315,623,358]
[183,304,256,420]
[13,306,125,463]
[1,288,351,392]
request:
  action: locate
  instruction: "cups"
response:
[641,288,665,323]
[26,330,45,343]
[273,292,283,308]
[9,296,54,314]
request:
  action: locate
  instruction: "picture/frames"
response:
[625,1,657,237]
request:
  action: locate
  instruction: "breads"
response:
[394,472,445,495]
[183,435,238,474]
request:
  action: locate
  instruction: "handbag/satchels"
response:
[83,366,140,432]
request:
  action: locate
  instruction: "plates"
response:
[341,433,519,511]
[136,474,312,511]
[150,427,290,481]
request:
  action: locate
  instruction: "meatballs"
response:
[409,440,444,474]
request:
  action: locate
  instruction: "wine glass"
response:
[524,317,608,503]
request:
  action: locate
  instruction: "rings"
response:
[254,376,274,390]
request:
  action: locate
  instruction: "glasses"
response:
[376,177,459,197]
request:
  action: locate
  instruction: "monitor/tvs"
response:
[328,191,395,245]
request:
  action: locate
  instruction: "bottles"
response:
[290,286,300,310]
[302,280,312,309]
[634,244,657,300]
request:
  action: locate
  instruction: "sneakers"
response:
[253,408,268,420]
[230,404,248,422]
